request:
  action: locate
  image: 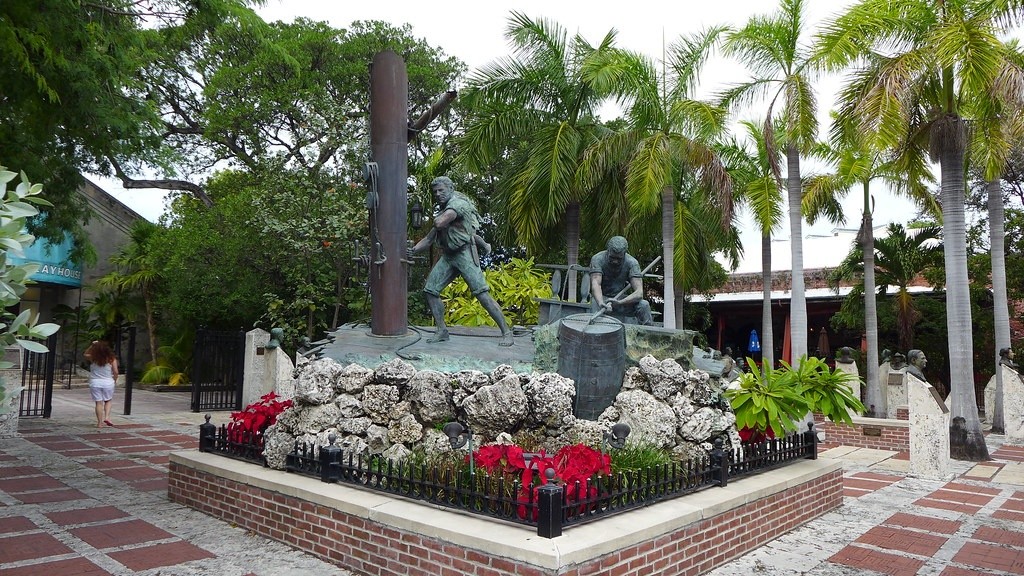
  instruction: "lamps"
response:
[442,423,463,443]
[613,424,630,446]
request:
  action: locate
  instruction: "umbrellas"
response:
[749,330,761,359]
[818,327,829,355]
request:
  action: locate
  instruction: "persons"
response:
[591,236,653,326]
[84,341,118,427]
[407,176,513,346]
[837,347,854,363]
[882,349,891,363]
[905,350,927,382]
[998,347,1020,371]
[265,328,284,349]
[298,337,313,358]
[724,347,733,363]
[734,357,744,373]
[712,349,722,360]
[894,353,906,370]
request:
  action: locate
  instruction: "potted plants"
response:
[725,356,867,450]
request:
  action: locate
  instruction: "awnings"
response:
[6,205,83,289]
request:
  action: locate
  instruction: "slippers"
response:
[104,420,114,426]
